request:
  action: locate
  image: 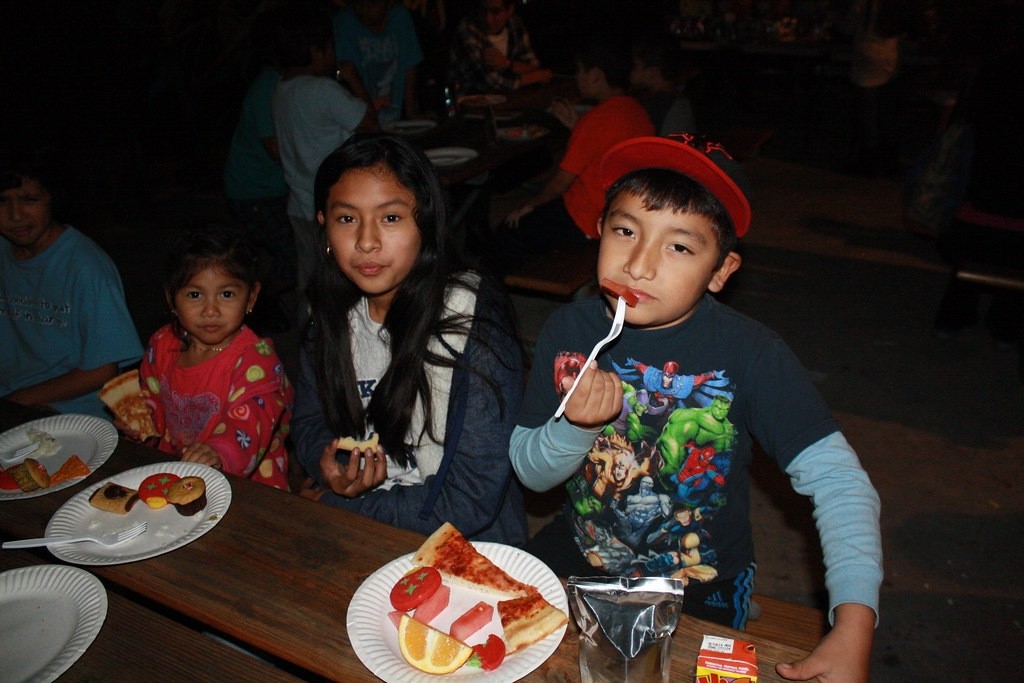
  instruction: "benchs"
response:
[509,532,822,654]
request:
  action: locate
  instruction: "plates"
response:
[0,564,109,683]
[495,125,548,142]
[346,541,572,683]
[425,150,478,168]
[45,462,233,566]
[457,94,506,106]
[1,414,119,505]
[383,120,435,136]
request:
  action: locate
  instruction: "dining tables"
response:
[392,95,555,260]
[0,397,824,683]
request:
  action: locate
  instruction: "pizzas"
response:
[497,594,571,651]
[411,521,537,594]
[336,432,379,455]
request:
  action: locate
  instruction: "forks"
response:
[2,441,40,464]
[1,519,147,550]
[553,296,626,421]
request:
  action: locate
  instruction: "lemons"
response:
[399,615,474,675]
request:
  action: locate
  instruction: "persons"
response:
[667,0,1024,349]
[331,0,423,127]
[456,1,553,97]
[139,228,293,495]
[628,40,695,142]
[0,150,143,431]
[505,129,885,682]
[267,0,382,337]
[286,134,527,552]
[504,47,657,273]
[223,20,287,227]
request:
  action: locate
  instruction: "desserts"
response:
[391,565,442,612]
[89,474,207,518]
[468,634,507,672]
[0,464,24,491]
[506,125,545,138]
[12,458,50,493]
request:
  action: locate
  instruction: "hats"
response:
[598,129,752,239]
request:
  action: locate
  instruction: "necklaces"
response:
[185,325,244,352]
